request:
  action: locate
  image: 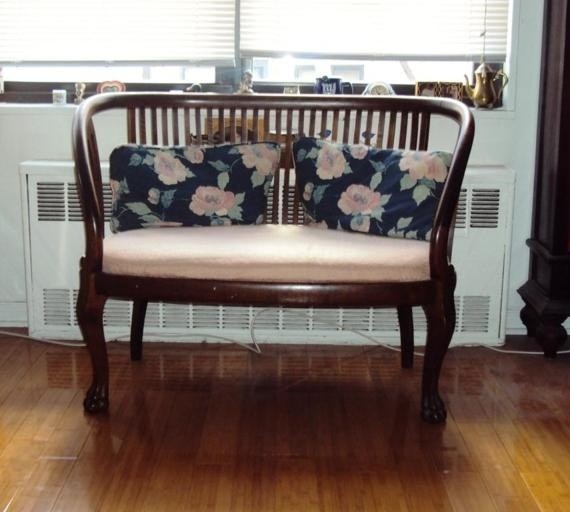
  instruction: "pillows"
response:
[109,140,282,234]
[292,136,454,243]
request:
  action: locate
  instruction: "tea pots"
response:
[463,58,509,110]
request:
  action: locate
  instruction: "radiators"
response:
[18,159,516,347]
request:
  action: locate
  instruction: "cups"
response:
[314,76,354,95]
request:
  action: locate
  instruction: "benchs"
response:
[72,90,476,424]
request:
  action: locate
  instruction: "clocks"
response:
[360,82,394,95]
